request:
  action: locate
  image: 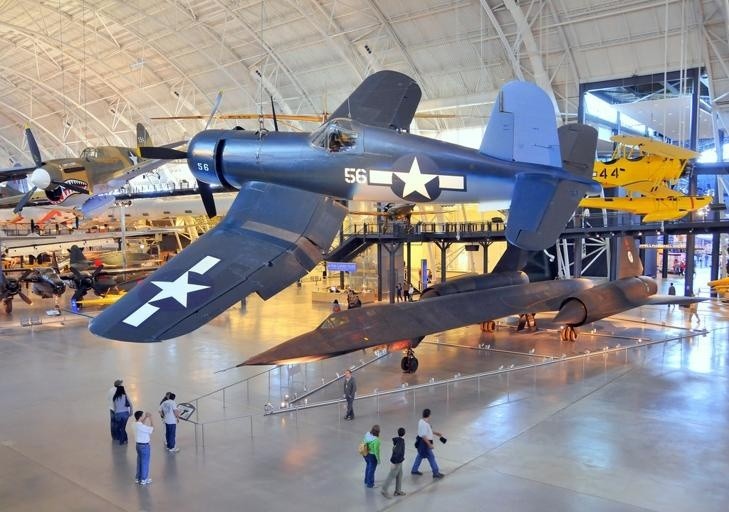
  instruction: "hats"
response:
[114,380,123,387]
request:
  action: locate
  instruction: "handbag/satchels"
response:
[359,443,368,456]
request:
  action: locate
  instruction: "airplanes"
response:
[577,136,713,223]
[0,123,188,312]
[215,237,728,374]
[88,70,601,342]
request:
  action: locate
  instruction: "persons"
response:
[112,385,132,444]
[687,294,701,323]
[411,407,443,479]
[360,423,381,488]
[160,393,181,453]
[159,391,171,447]
[342,369,357,421]
[331,280,415,312]
[379,426,408,498]
[108,378,126,440]
[674,258,687,276]
[132,409,153,484]
[667,282,676,309]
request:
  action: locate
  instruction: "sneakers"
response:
[167,447,179,452]
[394,492,406,496]
[411,471,422,475]
[433,474,444,479]
[136,479,153,485]
